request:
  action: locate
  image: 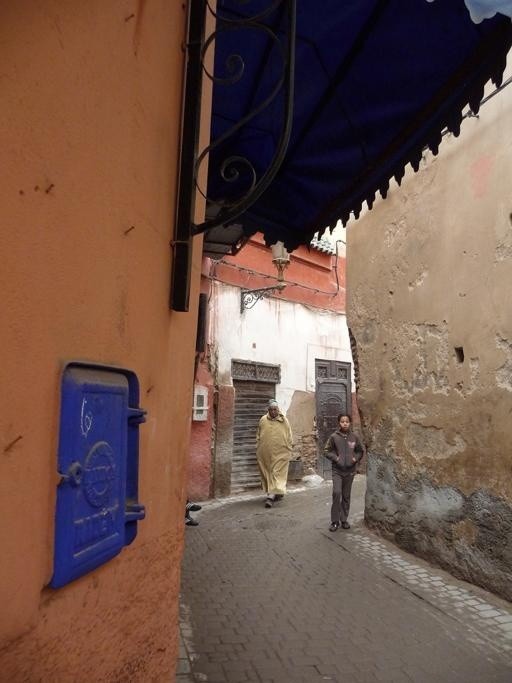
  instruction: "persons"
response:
[254,399,294,508]
[185,499,201,528]
[322,414,364,532]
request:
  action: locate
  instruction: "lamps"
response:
[241,232,292,310]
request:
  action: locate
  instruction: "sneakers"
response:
[185,501,201,511]
[185,515,199,526]
[266,494,284,507]
[341,521,350,529]
[330,521,339,531]
